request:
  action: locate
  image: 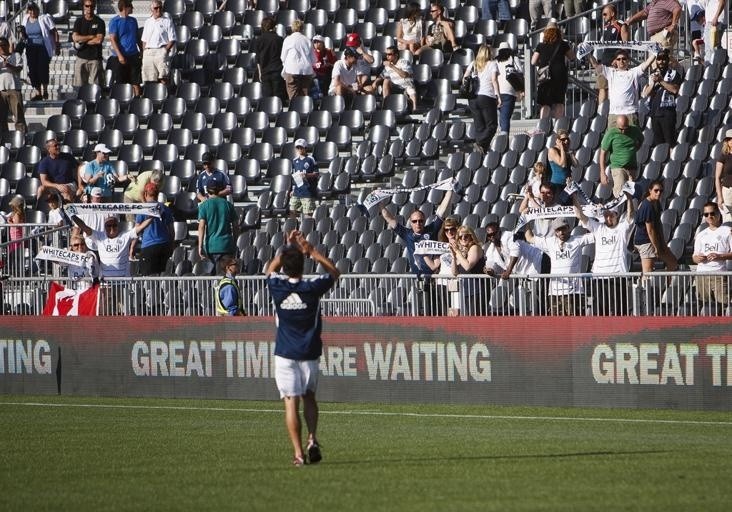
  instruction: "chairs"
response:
[0,0,732,315]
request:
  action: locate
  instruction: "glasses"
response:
[704,212,718,217]
[83,4,162,10]
[410,220,498,241]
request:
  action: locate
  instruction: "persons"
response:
[262,228,345,466]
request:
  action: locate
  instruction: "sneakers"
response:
[292,441,323,468]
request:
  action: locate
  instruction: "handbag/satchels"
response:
[457,75,478,99]
[535,65,551,87]
[505,65,524,92]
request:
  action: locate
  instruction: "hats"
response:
[27,2,38,9]
[723,128,732,142]
[91,186,104,198]
[544,22,561,30]
[93,143,113,154]
[104,216,119,225]
[294,138,308,150]
[551,216,568,229]
[497,41,510,51]
[690,10,705,21]
[312,33,361,59]
[46,193,58,202]
[656,50,669,60]
[603,205,619,217]
[201,152,213,165]
